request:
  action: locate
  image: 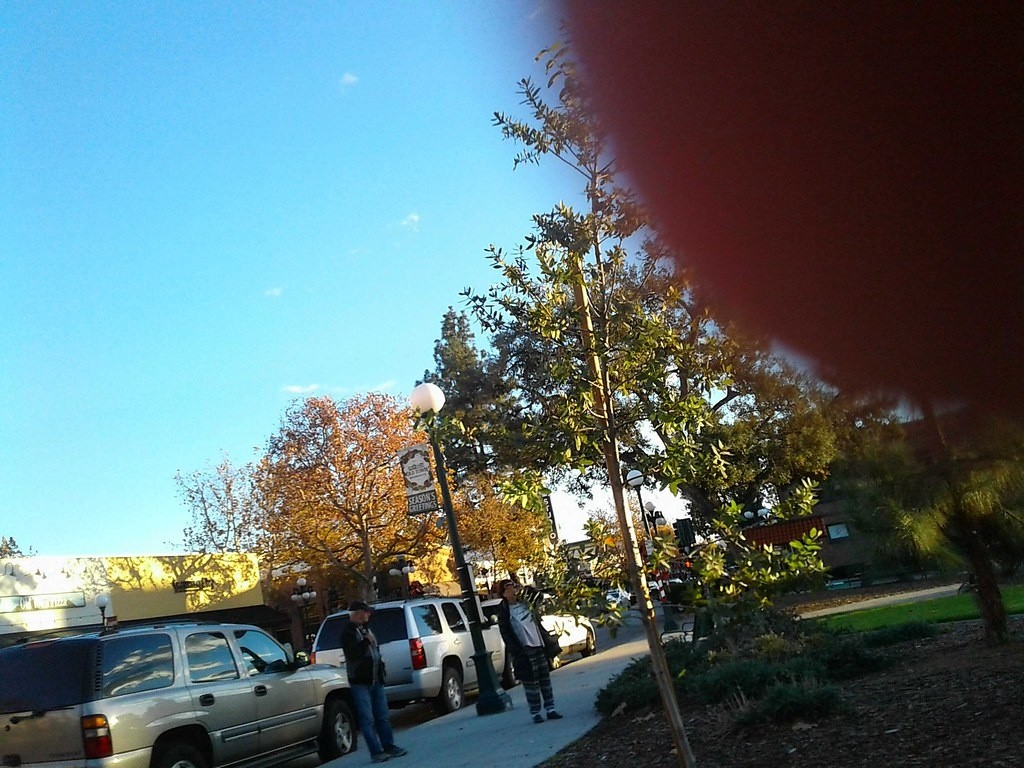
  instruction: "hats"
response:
[349,601,375,611]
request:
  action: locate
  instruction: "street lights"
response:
[96,594,109,626]
[625,470,655,541]
[641,501,667,537]
[290,578,318,648]
[388,555,417,589]
[410,383,512,717]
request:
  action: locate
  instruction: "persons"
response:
[340,601,408,763]
[497,579,564,723]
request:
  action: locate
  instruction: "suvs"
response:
[309,596,523,717]
[0,621,359,768]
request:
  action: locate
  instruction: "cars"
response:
[602,576,685,613]
[477,593,598,672]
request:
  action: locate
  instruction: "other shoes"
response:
[384,744,408,757]
[533,715,545,723]
[546,710,562,719]
[370,749,391,763]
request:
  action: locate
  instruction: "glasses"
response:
[505,583,514,587]
